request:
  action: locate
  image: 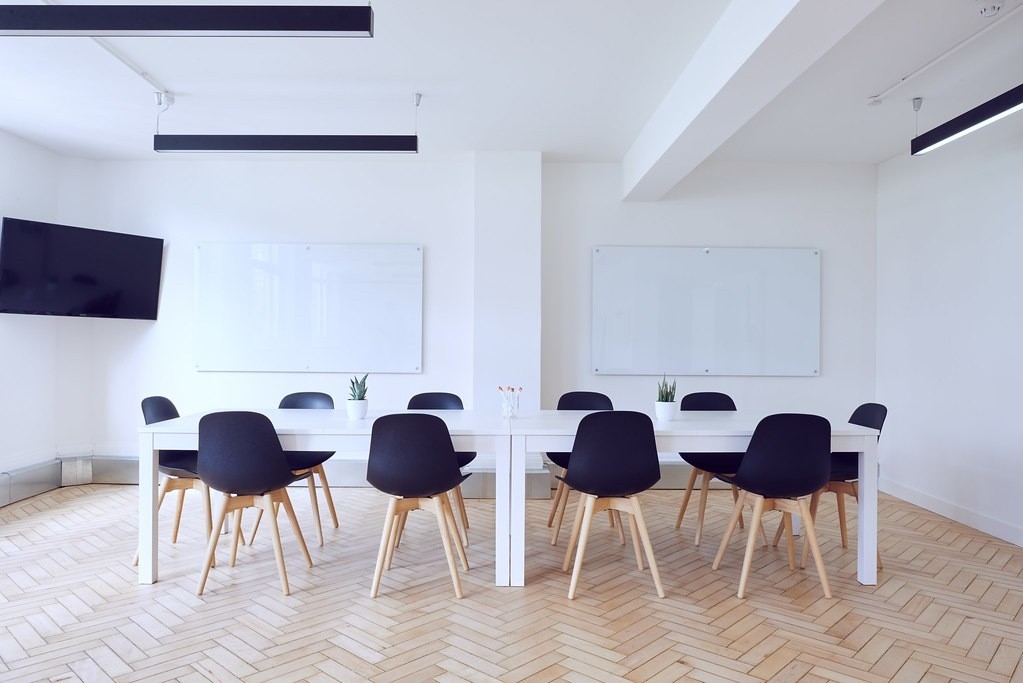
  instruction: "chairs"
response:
[367,413,472,602]
[197,410,313,603]
[565,409,668,604]
[133,391,888,574]
[713,411,834,600]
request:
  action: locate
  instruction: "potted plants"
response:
[655,373,678,423]
[346,372,369,421]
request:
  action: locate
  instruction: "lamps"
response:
[151,91,421,155]
[909,84,1023,156]
[0,0,375,37]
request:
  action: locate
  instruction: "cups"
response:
[502,394,519,419]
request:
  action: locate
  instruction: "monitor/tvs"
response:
[0,217,164,320]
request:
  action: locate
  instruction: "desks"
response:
[511,411,881,587]
[137,409,510,588]
